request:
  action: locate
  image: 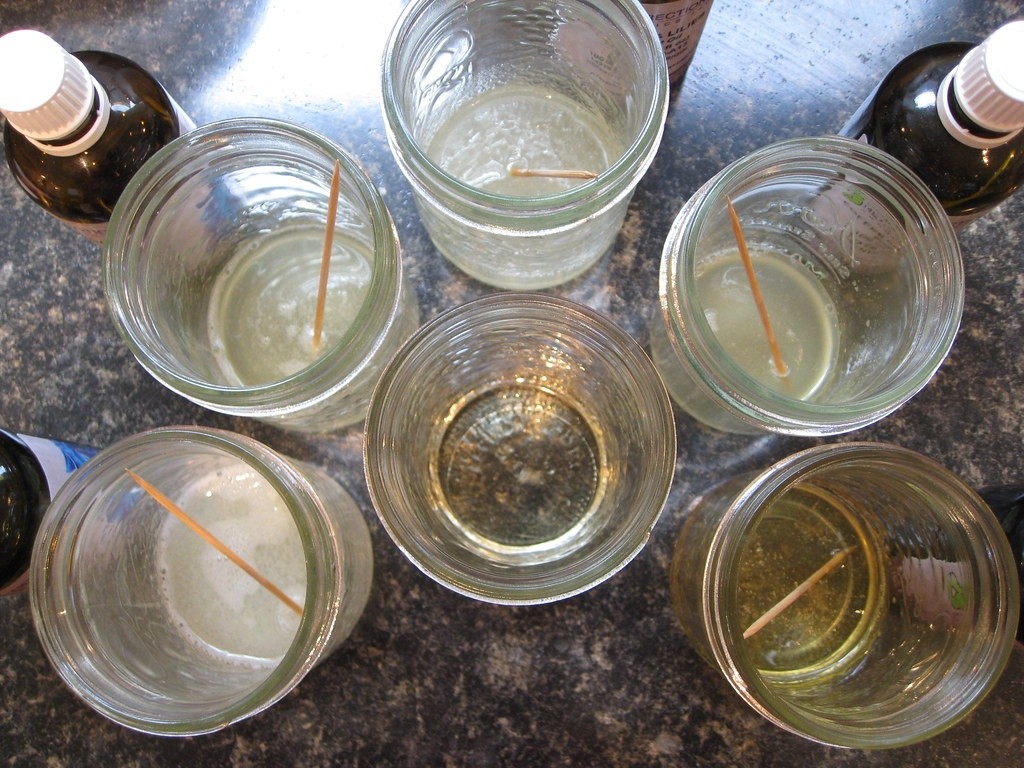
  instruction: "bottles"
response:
[785,20,1024,246]
[639,0,714,87]
[0,428,162,597]
[0,29,246,278]
[884,483,1024,649]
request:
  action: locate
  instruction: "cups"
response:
[668,441,1020,749]
[26,424,373,738]
[100,117,420,436]
[378,0,671,292]
[362,292,679,609]
[647,134,967,440]
